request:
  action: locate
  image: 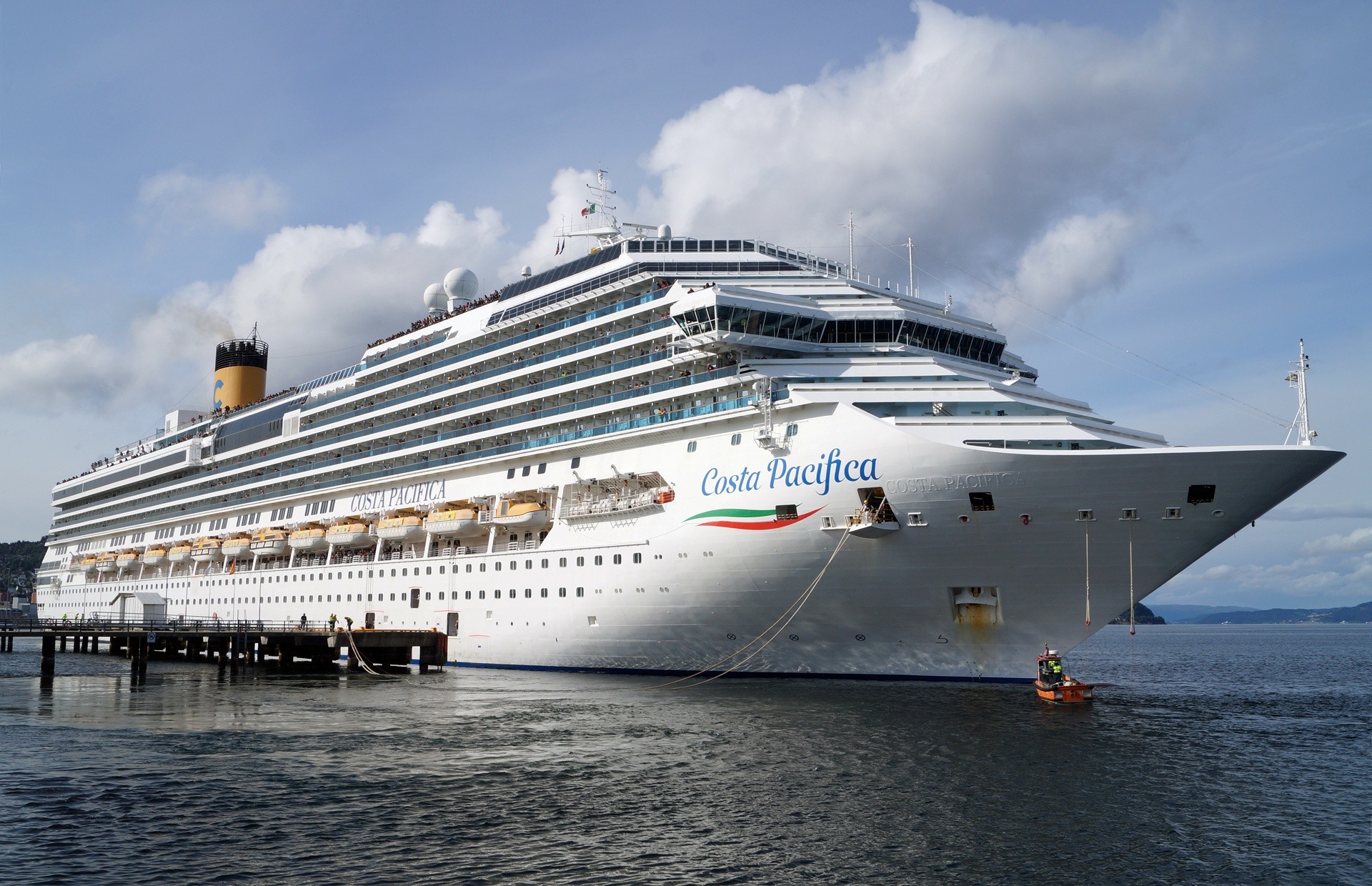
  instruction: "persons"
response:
[858,500,874,525]
[329,613,339,633]
[344,617,353,631]
[49,279,735,543]
[295,546,403,567]
[1052,661,1062,683]
[63,612,84,624]
[366,618,374,629]
[1050,660,1055,669]
[300,612,307,631]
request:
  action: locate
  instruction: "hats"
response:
[1055,661,1058,663]
[863,500,868,503]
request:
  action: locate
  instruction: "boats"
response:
[191,536,225,561]
[221,532,254,560]
[426,499,490,541]
[144,545,167,566]
[492,495,552,530]
[116,549,143,571]
[95,552,118,572]
[1034,643,1095,707]
[327,513,377,550]
[288,521,329,554]
[167,540,193,563]
[81,554,97,572]
[249,526,291,558]
[377,507,427,543]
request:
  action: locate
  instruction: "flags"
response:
[228,557,235,575]
[581,202,595,216]
[554,237,565,256]
[203,558,211,578]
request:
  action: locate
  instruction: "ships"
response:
[35,171,1350,681]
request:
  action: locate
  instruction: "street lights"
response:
[258,552,271,630]
[162,542,172,619]
[4,568,10,632]
[83,569,97,622]
